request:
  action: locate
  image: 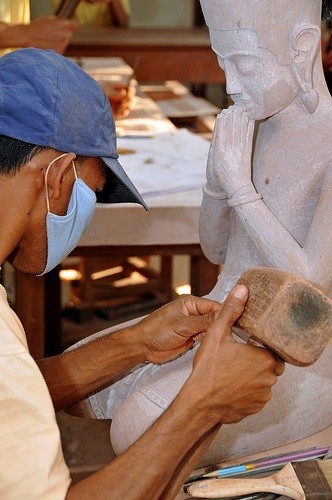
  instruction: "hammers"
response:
[151,264,332,500]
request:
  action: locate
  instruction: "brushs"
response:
[185,458,332,500]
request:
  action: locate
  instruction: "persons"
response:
[0,47,285,500]
[61,0,332,471]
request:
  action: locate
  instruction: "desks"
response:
[16,79,222,364]
[51,26,332,84]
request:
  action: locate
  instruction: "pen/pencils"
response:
[198,444,329,477]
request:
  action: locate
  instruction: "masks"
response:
[35,153,97,277]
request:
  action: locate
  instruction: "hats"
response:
[0,49,150,212]
